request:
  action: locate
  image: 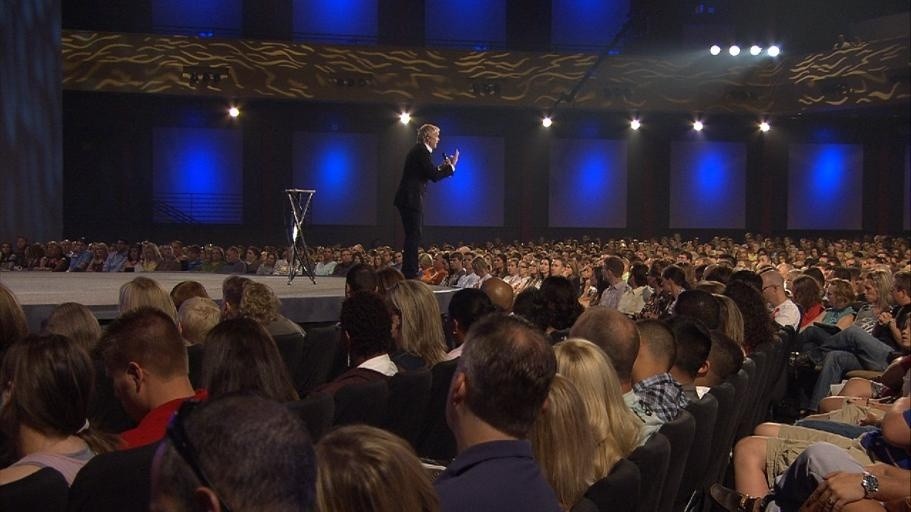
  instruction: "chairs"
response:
[271,323,435,432]
[544,326,794,511]
[184,344,203,385]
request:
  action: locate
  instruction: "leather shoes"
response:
[706,480,764,512]
[788,351,824,372]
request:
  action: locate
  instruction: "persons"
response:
[393,124,459,281]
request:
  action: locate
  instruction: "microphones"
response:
[442,153,448,160]
